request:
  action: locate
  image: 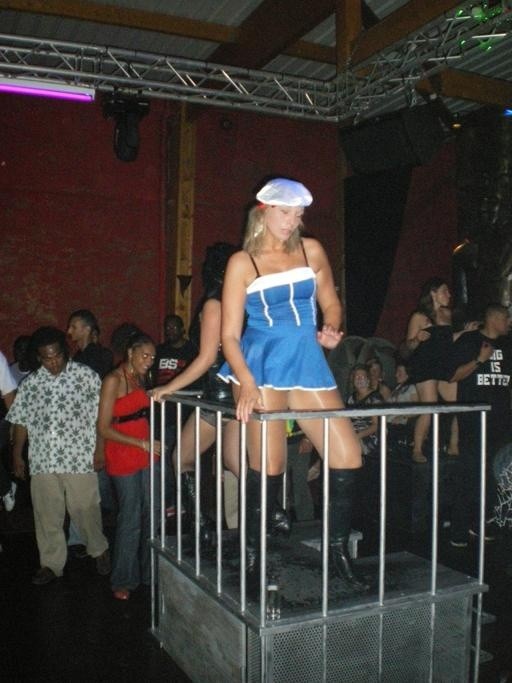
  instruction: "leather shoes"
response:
[111,590,131,601]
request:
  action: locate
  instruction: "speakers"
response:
[113,118,140,161]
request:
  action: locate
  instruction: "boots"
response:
[241,468,292,611]
[329,466,372,594]
[180,470,214,556]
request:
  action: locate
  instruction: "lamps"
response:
[0,75,95,102]
[339,61,472,179]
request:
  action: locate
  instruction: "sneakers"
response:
[31,566,57,586]
[448,524,498,549]
[95,549,112,576]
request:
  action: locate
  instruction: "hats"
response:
[255,177,314,207]
